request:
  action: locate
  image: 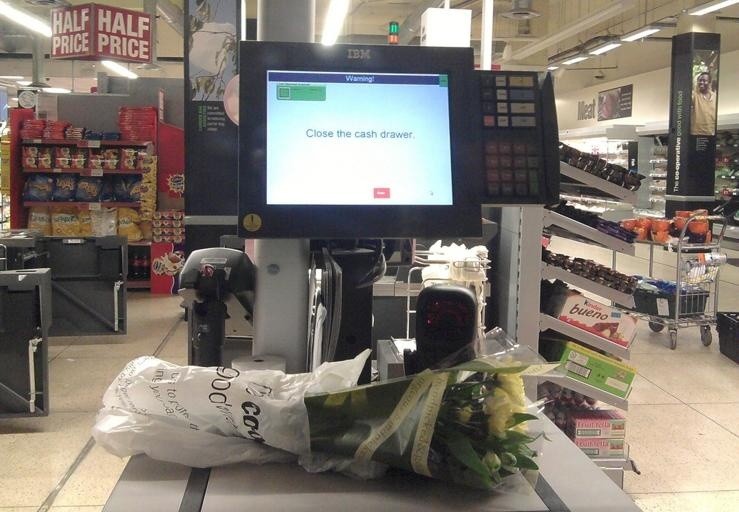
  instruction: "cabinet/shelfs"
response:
[558,118,739,251]
[7,105,159,296]
[499,156,639,490]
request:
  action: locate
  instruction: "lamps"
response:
[545,0,739,72]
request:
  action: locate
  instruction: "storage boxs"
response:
[573,440,627,460]
[566,409,629,440]
[619,287,712,320]
[548,280,639,351]
[540,335,639,399]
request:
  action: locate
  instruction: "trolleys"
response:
[610,213,729,348]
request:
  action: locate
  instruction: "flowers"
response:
[305,344,554,496]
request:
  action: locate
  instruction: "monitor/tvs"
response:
[236,40,483,241]
